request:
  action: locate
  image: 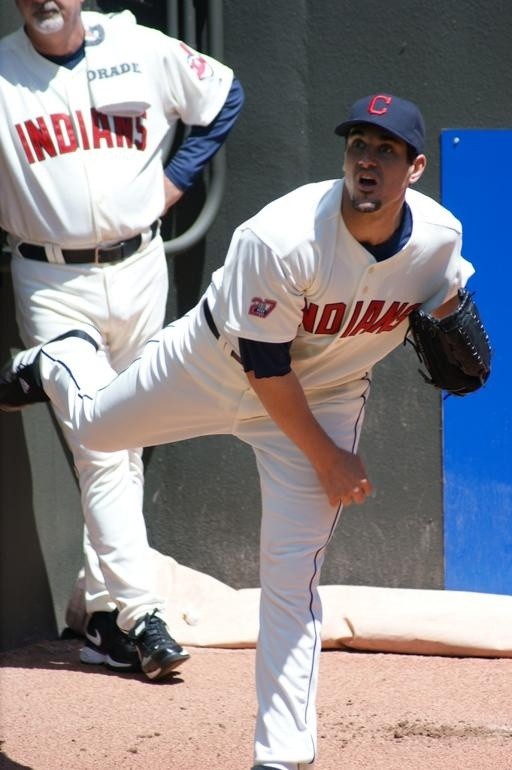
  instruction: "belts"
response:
[18,220,164,267]
[200,297,246,367]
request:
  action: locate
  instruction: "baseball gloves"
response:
[409,287,491,395]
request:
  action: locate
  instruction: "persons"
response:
[0,92,498,770]
[0,1,246,683]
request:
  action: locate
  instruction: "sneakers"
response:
[78,610,140,671]
[127,613,191,682]
[0,324,102,413]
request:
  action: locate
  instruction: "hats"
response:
[334,94,427,157]
